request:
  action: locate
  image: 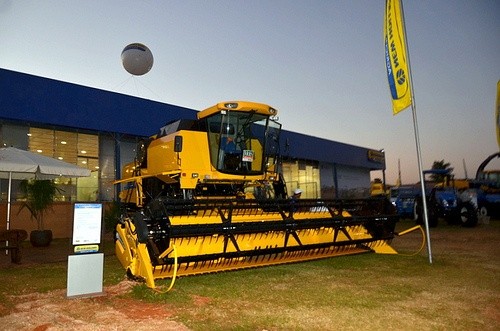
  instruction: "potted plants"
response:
[20,174,59,246]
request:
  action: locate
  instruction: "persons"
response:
[457,187,462,199]
[290,189,303,199]
[461,187,470,203]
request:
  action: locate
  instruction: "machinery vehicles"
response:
[110,101,425,293]
[389,152,500,228]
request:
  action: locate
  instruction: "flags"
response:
[383,0,412,116]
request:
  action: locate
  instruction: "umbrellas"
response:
[0,146,91,257]
